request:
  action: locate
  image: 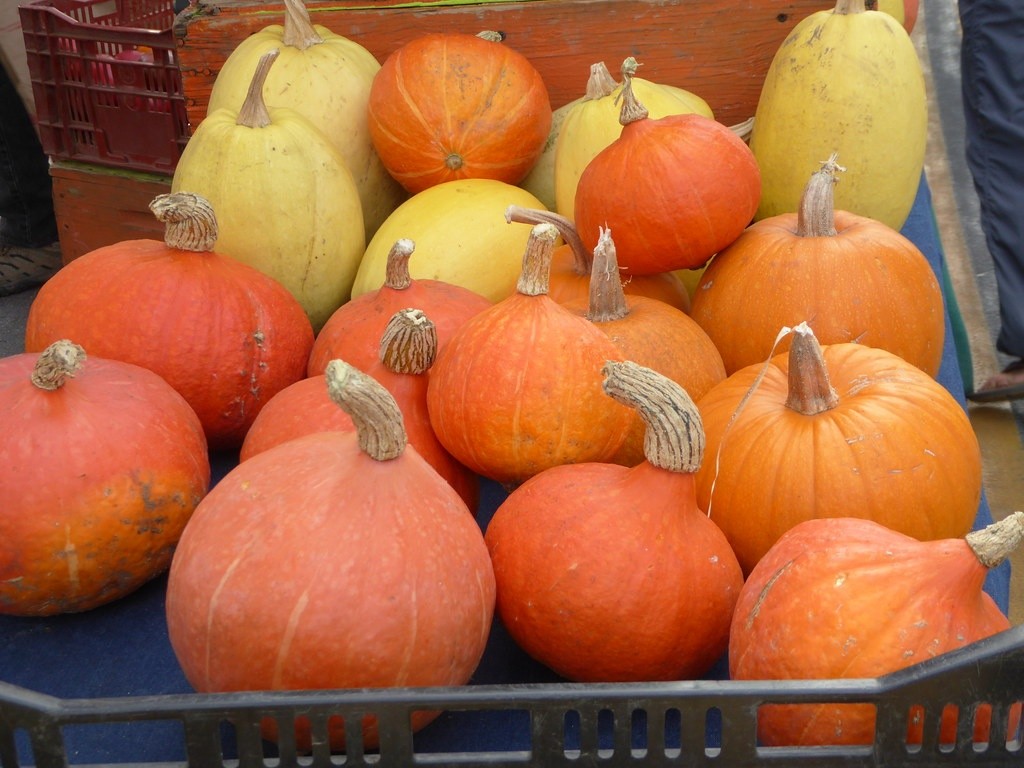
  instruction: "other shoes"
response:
[0,244,64,296]
[968,369,1024,402]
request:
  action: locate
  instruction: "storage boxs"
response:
[44,157,173,266]
[17,0,191,174]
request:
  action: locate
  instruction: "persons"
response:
[959,0,1023,402]
[0,39,60,295]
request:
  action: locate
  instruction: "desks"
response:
[0,175,1012,768]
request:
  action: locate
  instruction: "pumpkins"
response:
[0,0,1023,748]
